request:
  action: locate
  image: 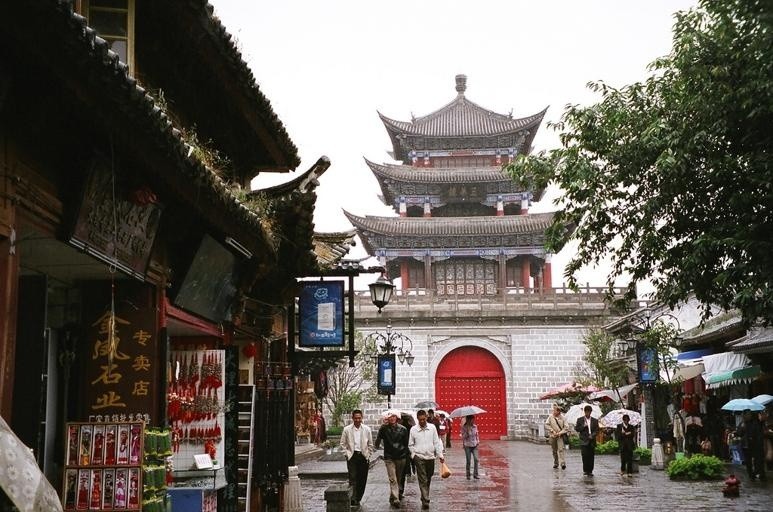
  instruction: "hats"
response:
[381,409,402,421]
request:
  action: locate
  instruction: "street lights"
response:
[618,307,680,384]
[364,325,415,409]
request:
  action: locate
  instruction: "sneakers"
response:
[751,474,765,481]
[562,465,566,470]
[389,495,404,506]
[621,471,624,475]
[422,501,429,509]
[474,476,480,479]
[584,472,588,475]
[589,473,593,476]
[468,476,470,480]
[553,464,558,468]
[628,473,632,477]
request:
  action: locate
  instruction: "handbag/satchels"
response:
[563,433,569,444]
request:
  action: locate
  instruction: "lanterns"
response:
[242,344,255,364]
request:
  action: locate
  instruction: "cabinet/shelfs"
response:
[237,384,256,512]
[61,421,145,512]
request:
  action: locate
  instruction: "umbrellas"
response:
[449,405,487,418]
[601,409,641,429]
[721,398,765,423]
[565,403,604,425]
[414,401,440,409]
[539,382,612,402]
[751,394,773,406]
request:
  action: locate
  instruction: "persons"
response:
[545,406,569,470]
[617,415,635,477]
[574,405,599,476]
[736,409,769,482]
[340,409,480,509]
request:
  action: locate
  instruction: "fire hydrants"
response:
[722,475,741,497]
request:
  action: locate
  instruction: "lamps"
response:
[360,265,395,314]
